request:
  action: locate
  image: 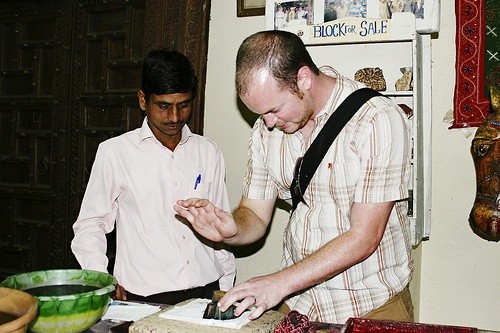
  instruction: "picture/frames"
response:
[236,0,265,18]
[264,0,440,33]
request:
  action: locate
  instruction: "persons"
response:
[69,49,237,305]
[173,30,414,325]
[274,0,424,28]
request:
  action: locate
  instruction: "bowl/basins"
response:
[0,287,38,333]
[0,269,118,333]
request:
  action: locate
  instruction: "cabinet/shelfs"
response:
[285,12,431,246]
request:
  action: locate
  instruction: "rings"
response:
[249,295,256,304]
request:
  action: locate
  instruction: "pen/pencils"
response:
[194,174,201,189]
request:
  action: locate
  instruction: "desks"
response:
[82,300,500,333]
